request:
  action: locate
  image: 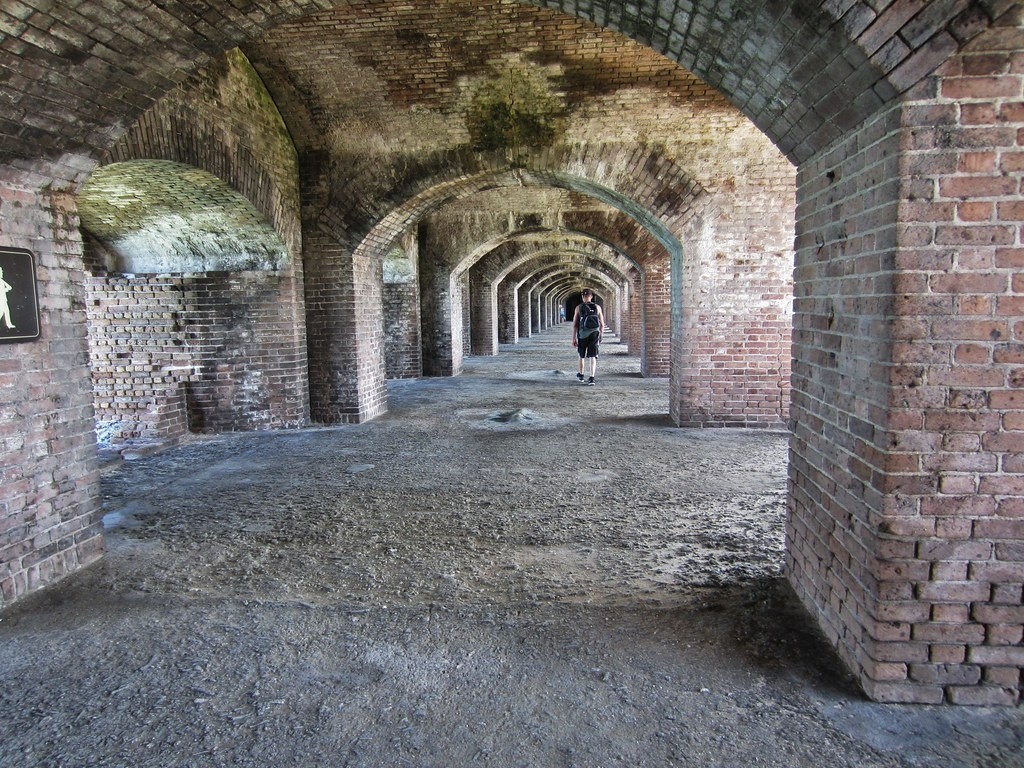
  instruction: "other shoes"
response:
[588,377,595,385]
[576,373,584,382]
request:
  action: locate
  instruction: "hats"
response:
[581,289,592,296]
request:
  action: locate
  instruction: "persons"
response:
[571,289,604,386]
[559,305,566,322]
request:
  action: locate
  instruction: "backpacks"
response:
[580,302,599,328]
